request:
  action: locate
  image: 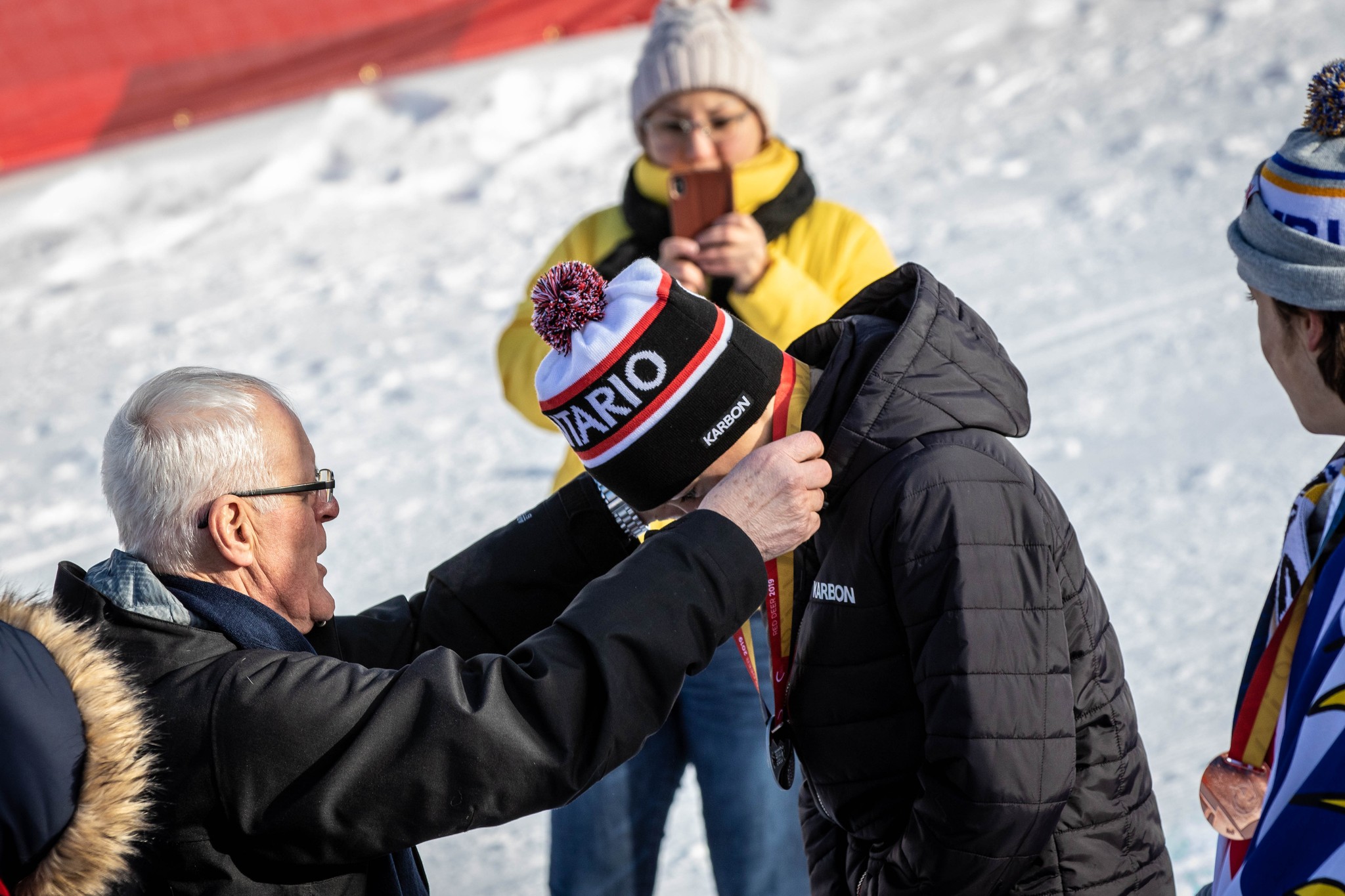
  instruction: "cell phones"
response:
[669,167,733,281]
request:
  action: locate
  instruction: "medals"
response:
[1200,751,1271,840]
[766,714,795,791]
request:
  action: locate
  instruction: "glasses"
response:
[196,469,335,529]
[641,107,751,146]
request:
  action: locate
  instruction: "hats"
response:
[529,255,785,513]
[628,0,772,145]
[1226,58,1345,309]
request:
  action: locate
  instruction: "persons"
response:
[1211,57,1345,896]
[532,258,1175,896]
[51,367,832,896]
[496,0,896,896]
[0,591,158,896]
